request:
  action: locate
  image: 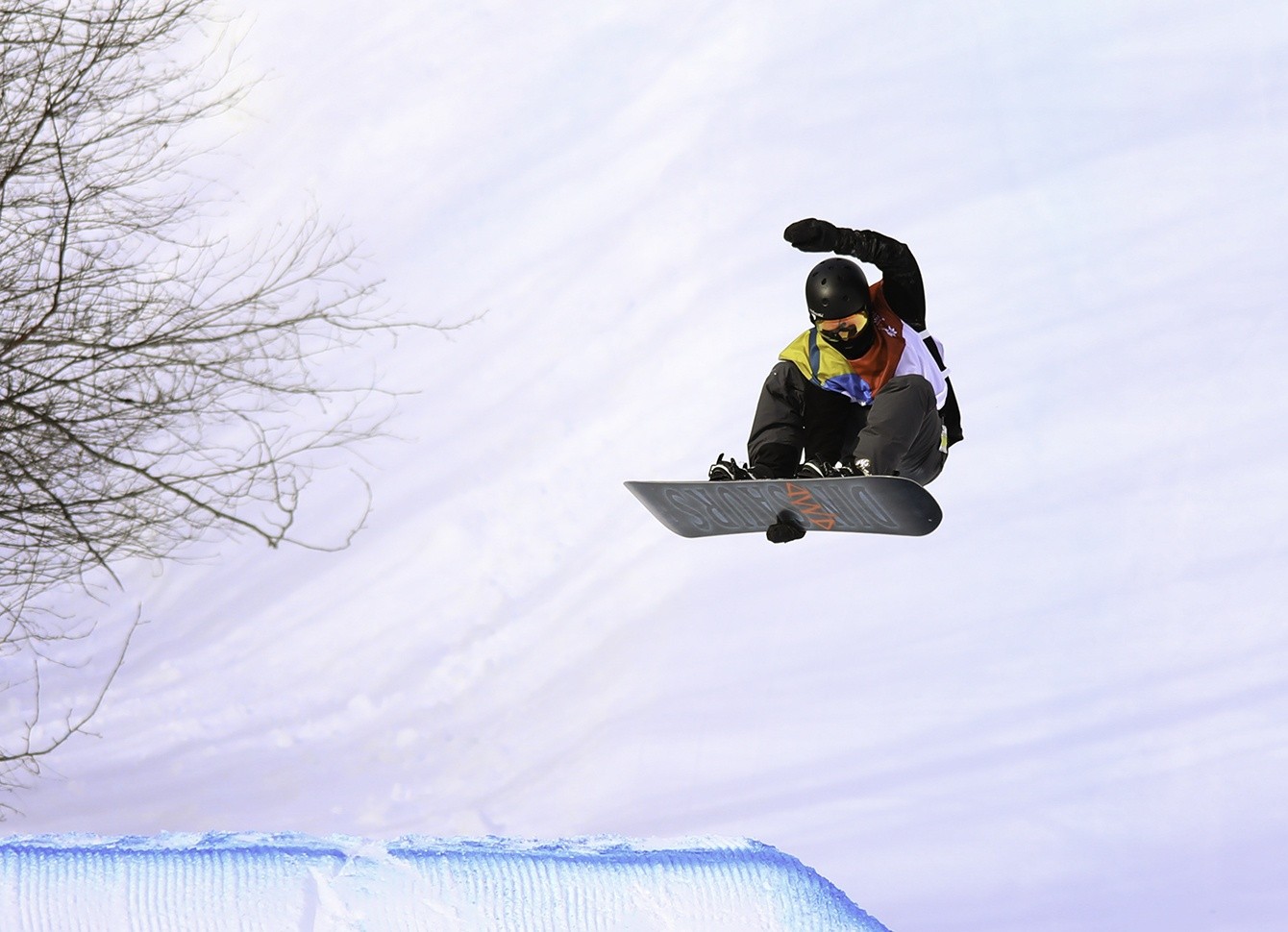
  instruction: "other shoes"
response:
[711,462,755,480]
[797,460,867,480]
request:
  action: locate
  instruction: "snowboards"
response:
[622,473,945,537]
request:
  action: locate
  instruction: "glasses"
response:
[814,312,871,341]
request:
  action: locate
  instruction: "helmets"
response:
[806,258,869,321]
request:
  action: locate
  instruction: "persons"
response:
[708,218,964,544]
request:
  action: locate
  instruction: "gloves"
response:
[784,218,856,255]
[767,522,806,543]
